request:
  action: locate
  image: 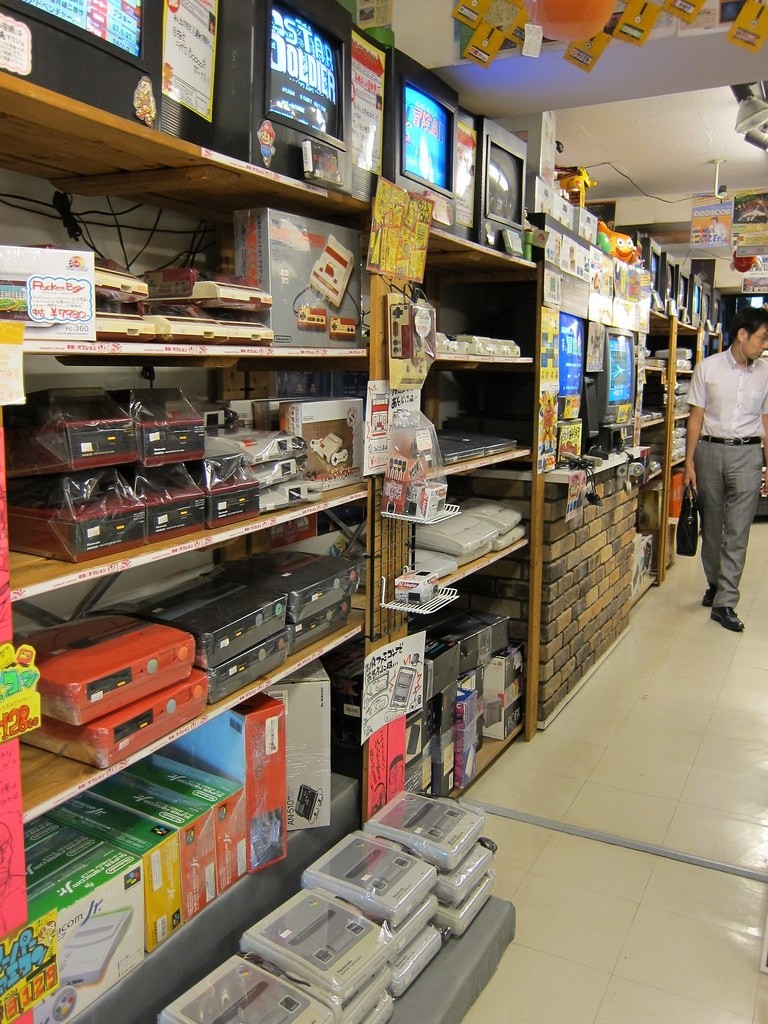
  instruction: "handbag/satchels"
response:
[676,482,698,558]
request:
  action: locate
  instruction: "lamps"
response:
[730,84,768,134]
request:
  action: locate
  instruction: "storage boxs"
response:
[638,479,663,531]
[262,660,332,832]
[555,193,575,231]
[573,207,598,245]
[216,397,363,492]
[491,111,557,191]
[24,657,286,1024]
[232,207,363,348]
[525,175,555,218]
[319,609,527,795]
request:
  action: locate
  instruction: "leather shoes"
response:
[710,606,745,631]
[702,583,718,606]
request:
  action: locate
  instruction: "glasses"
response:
[746,332,768,343]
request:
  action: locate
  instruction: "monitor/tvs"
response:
[557,237,768,425]
[0,0,528,261]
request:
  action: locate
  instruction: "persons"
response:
[682,307,768,631]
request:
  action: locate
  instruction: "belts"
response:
[702,435,761,446]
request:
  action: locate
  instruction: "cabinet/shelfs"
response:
[389,227,545,800]
[0,70,392,959]
[661,316,723,583]
[630,309,676,610]
